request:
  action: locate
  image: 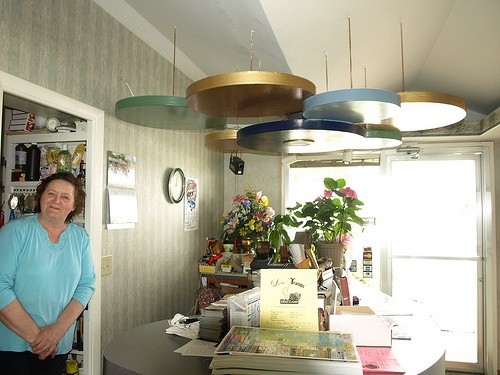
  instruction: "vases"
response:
[313,240,343,267]
[241,235,255,254]
[255,240,271,260]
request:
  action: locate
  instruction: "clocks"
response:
[167,167,186,204]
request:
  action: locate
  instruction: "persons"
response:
[0,173,97,375]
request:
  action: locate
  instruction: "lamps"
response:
[115,16,468,167]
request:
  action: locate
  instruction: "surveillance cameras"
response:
[229,156,244,175]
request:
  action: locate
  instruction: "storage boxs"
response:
[229,255,242,273]
[9,109,30,130]
[227,287,261,331]
[325,280,392,347]
[198,265,218,273]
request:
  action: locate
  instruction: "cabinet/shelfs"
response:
[4,131,86,225]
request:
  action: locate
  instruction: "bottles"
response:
[25,142,41,180]
[15,142,27,172]
[57,144,72,173]
[11,169,22,182]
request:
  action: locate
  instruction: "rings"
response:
[44,346,48,350]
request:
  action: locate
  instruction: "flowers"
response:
[294,176,366,254]
[219,181,276,244]
